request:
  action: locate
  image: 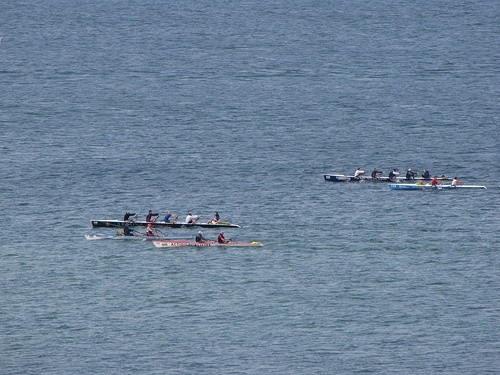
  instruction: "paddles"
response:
[126,214,137,223]
[133,230,147,242]
[187,217,199,230]
[170,216,179,222]
[223,237,233,242]
[155,229,169,240]
[150,215,159,222]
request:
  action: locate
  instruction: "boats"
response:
[323,174,454,182]
[83,234,216,241]
[90,219,242,228]
[388,184,487,190]
[152,238,264,249]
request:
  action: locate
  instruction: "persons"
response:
[124,223,134,236]
[355,167,365,178]
[124,211,137,222]
[422,169,429,178]
[218,230,229,245]
[389,169,399,178]
[371,168,382,178]
[432,176,459,186]
[196,231,207,243]
[146,223,156,236]
[186,212,198,224]
[211,212,220,225]
[406,168,418,179]
[164,211,177,223]
[146,210,159,222]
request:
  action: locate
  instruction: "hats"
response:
[220,231,224,235]
[198,231,203,235]
